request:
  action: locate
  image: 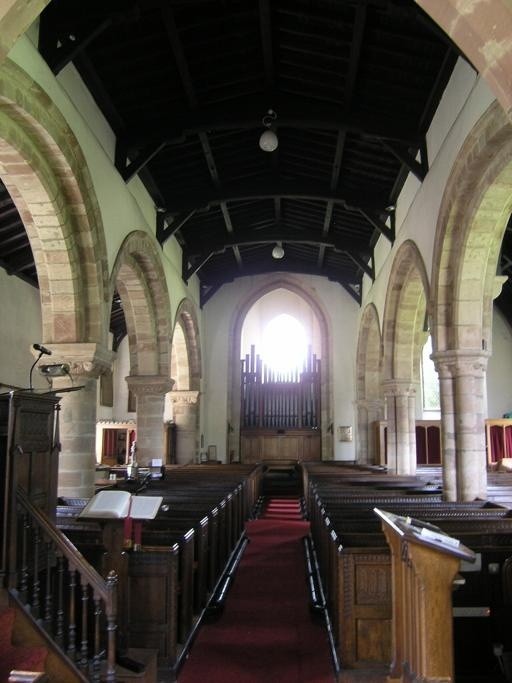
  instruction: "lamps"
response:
[259,108,280,151]
[272,240,285,259]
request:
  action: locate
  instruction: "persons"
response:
[116,434,126,465]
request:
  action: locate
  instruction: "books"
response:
[420,526,460,547]
[76,490,164,522]
[406,516,441,533]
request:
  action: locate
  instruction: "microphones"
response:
[33,344,52,355]
